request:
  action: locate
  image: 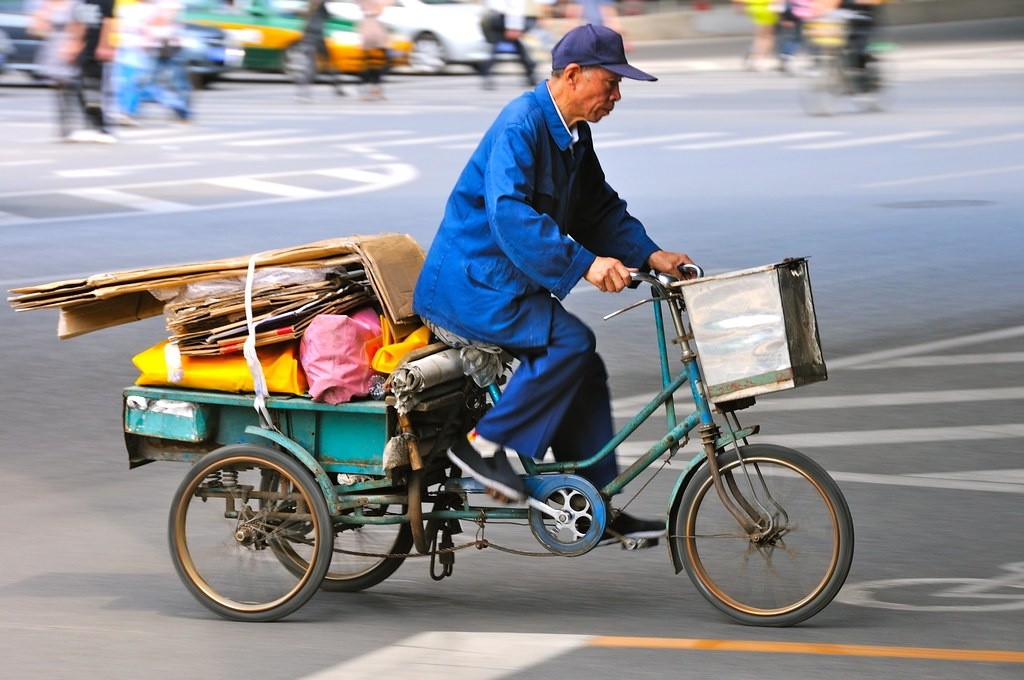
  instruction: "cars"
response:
[0,1,537,78]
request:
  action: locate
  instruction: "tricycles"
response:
[119,255,856,629]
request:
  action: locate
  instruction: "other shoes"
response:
[599,511,667,545]
[446,434,522,501]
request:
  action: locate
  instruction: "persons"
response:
[746,0,879,98]
[356,4,388,105]
[411,23,693,545]
[292,0,347,97]
[24,0,190,142]
[481,0,536,86]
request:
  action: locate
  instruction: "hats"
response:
[551,23,658,82]
